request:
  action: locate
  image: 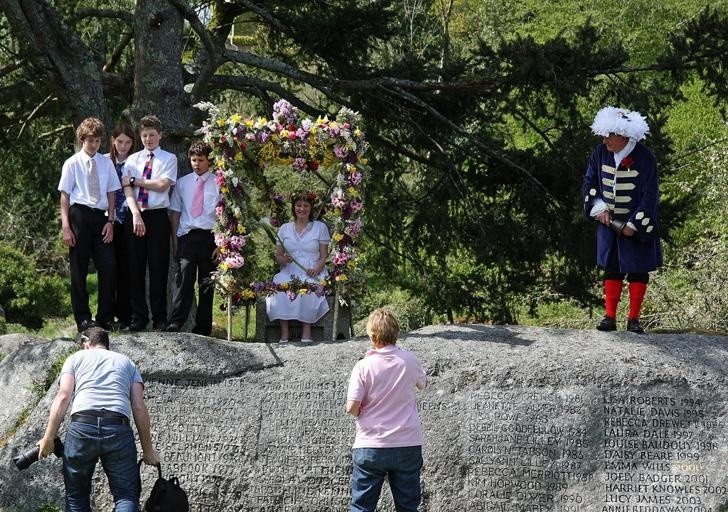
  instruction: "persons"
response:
[347,310,427,511]
[265,190,331,343]
[102,124,136,330]
[121,116,179,333]
[168,142,222,336]
[57,117,124,332]
[582,107,663,333]
[37,326,160,511]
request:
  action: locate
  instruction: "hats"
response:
[591,105,650,140]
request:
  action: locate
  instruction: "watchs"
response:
[108,220,115,225]
[129,176,135,185]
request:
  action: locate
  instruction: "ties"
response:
[137,154,152,213]
[190,177,204,219]
[84,159,101,204]
[114,163,129,224]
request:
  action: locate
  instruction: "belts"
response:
[70,412,129,424]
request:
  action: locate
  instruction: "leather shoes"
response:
[78,318,180,333]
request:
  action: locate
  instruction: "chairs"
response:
[193,97,356,343]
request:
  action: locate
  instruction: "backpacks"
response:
[132,457,190,511]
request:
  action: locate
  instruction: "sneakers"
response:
[596,317,617,331]
[628,319,645,333]
